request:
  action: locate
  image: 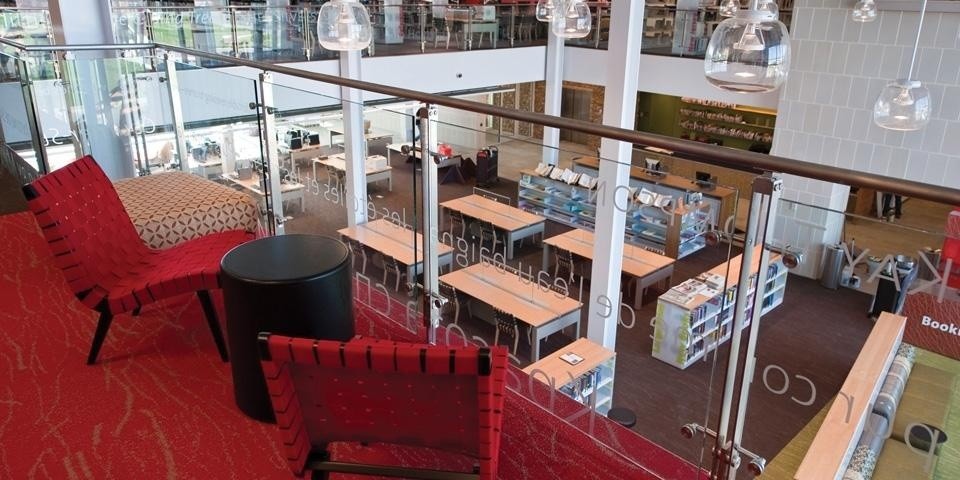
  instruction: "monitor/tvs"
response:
[695,171,711,182]
[597,146,601,158]
[645,157,661,171]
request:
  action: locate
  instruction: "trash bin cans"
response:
[917,250,941,281]
[821,244,845,289]
[477,145,498,188]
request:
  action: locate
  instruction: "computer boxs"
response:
[238,167,249,180]
[259,179,270,192]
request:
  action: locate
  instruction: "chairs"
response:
[336,187,666,355]
[256,332,509,480]
[21,154,258,364]
[375,8,539,49]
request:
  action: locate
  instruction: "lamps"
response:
[536,0,592,39]
[703,0,795,96]
[852,0,878,22]
[317,0,374,51]
[873,0,932,131]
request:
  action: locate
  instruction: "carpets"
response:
[750,340,960,479]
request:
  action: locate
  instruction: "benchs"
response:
[870,438,938,480]
[892,361,958,456]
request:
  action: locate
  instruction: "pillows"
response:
[842,411,888,479]
[874,341,917,422]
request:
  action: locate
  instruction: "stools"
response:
[108,170,260,249]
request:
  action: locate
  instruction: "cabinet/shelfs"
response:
[770,172,851,280]
[519,337,617,419]
[678,99,777,144]
[651,245,789,371]
[517,154,738,262]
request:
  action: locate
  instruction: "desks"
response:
[186,119,465,214]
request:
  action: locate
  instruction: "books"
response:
[519,161,711,267]
[665,258,778,361]
[142,1,795,59]
